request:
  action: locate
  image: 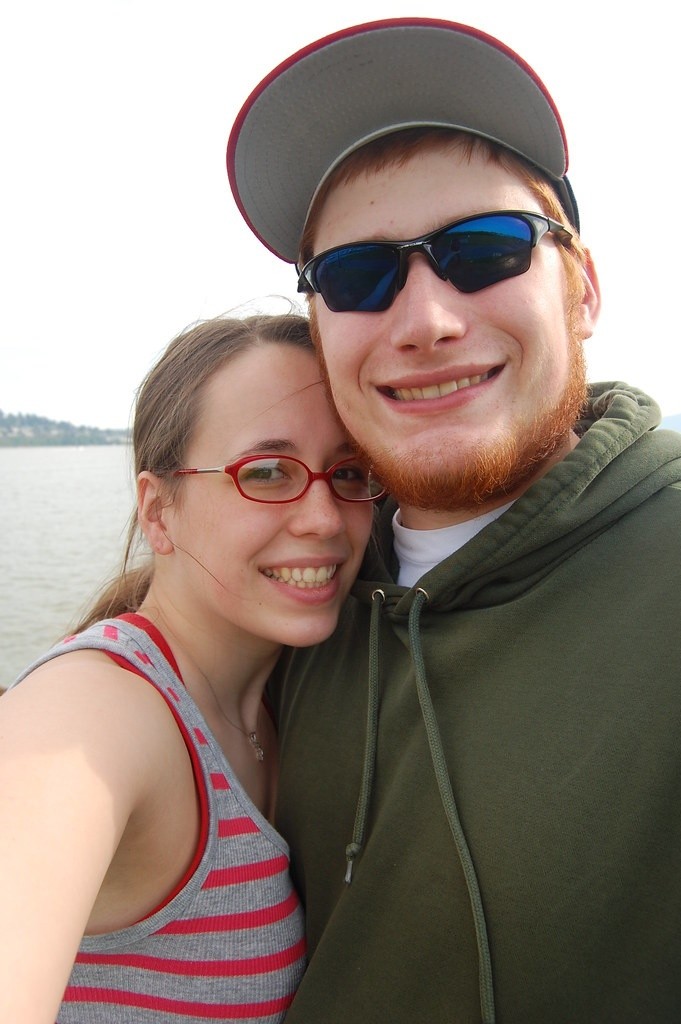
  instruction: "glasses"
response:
[164,454,389,504]
[297,209,574,313]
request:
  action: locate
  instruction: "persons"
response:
[226,15,681,1024]
[1,312,387,1024]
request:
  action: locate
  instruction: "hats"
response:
[227,16,580,264]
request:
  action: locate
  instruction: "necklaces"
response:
[155,611,270,763]
